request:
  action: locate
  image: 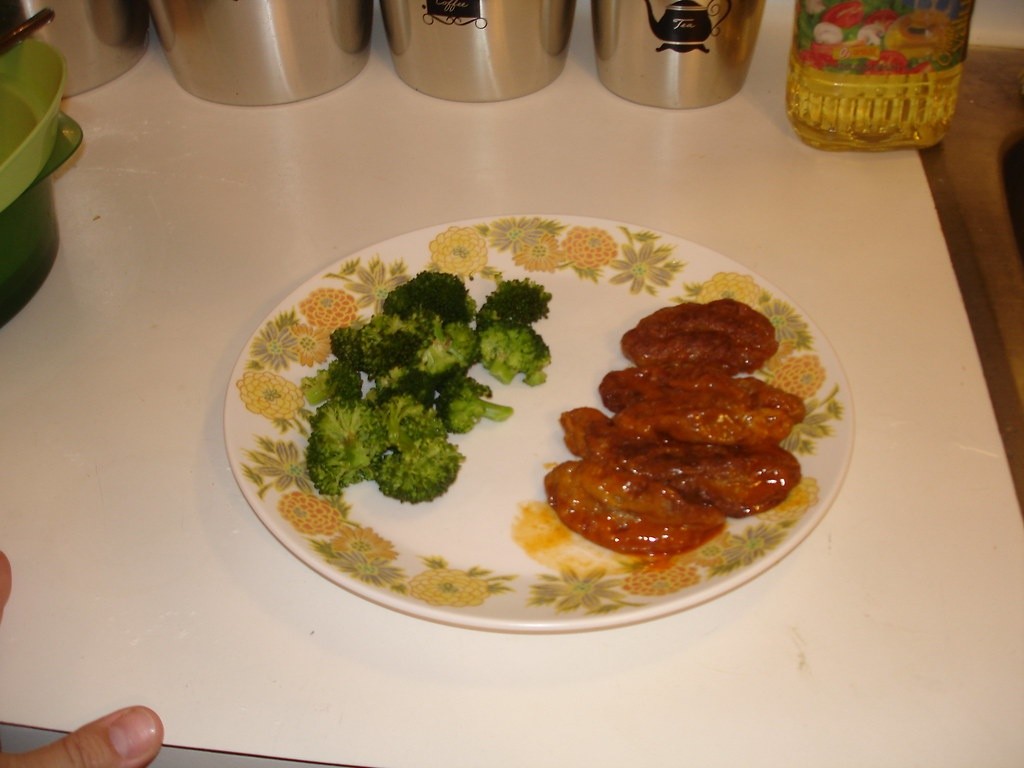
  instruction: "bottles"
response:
[786,1,973,153]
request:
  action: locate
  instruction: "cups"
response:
[0,0,148,101]
[147,1,375,109]
[591,0,767,111]
[378,0,576,104]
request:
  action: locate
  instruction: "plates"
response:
[223,214,853,634]
[0,38,67,213]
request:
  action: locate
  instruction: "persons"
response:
[0,552,162,768]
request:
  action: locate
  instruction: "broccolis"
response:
[303,269,553,504]
